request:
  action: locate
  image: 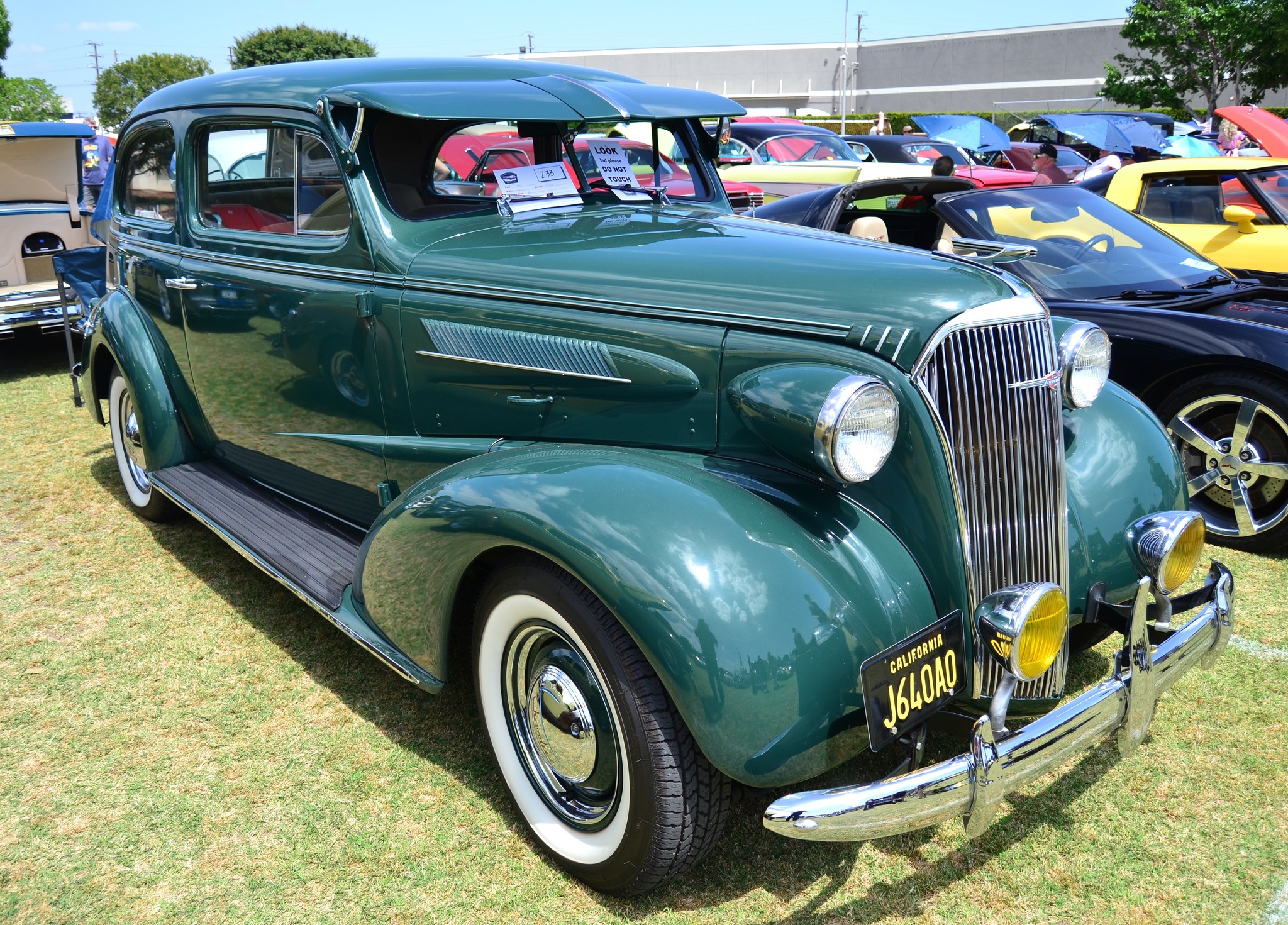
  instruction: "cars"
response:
[969,156,1287,281]
[85,54,1239,903]
[736,172,1287,562]
[0,82,1288,346]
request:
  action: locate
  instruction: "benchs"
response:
[144,182,425,223]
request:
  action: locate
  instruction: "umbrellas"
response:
[1038,114,1227,182]
[910,115,1012,152]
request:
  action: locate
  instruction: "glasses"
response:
[90,127,97,130]
[1032,154,1048,159]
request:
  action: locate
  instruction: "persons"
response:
[1217,118,1250,157]
[903,125,917,154]
[1067,148,1122,184]
[896,155,954,210]
[720,129,730,144]
[433,157,450,182]
[1029,143,1069,185]
[853,144,869,154]
[869,111,892,135]
[81,117,114,209]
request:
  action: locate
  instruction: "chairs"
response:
[1182,193,1217,223]
[843,216,889,243]
[1143,196,1173,222]
[931,208,978,257]
[209,203,294,233]
[52,246,107,406]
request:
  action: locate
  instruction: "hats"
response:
[1029,144,1058,159]
